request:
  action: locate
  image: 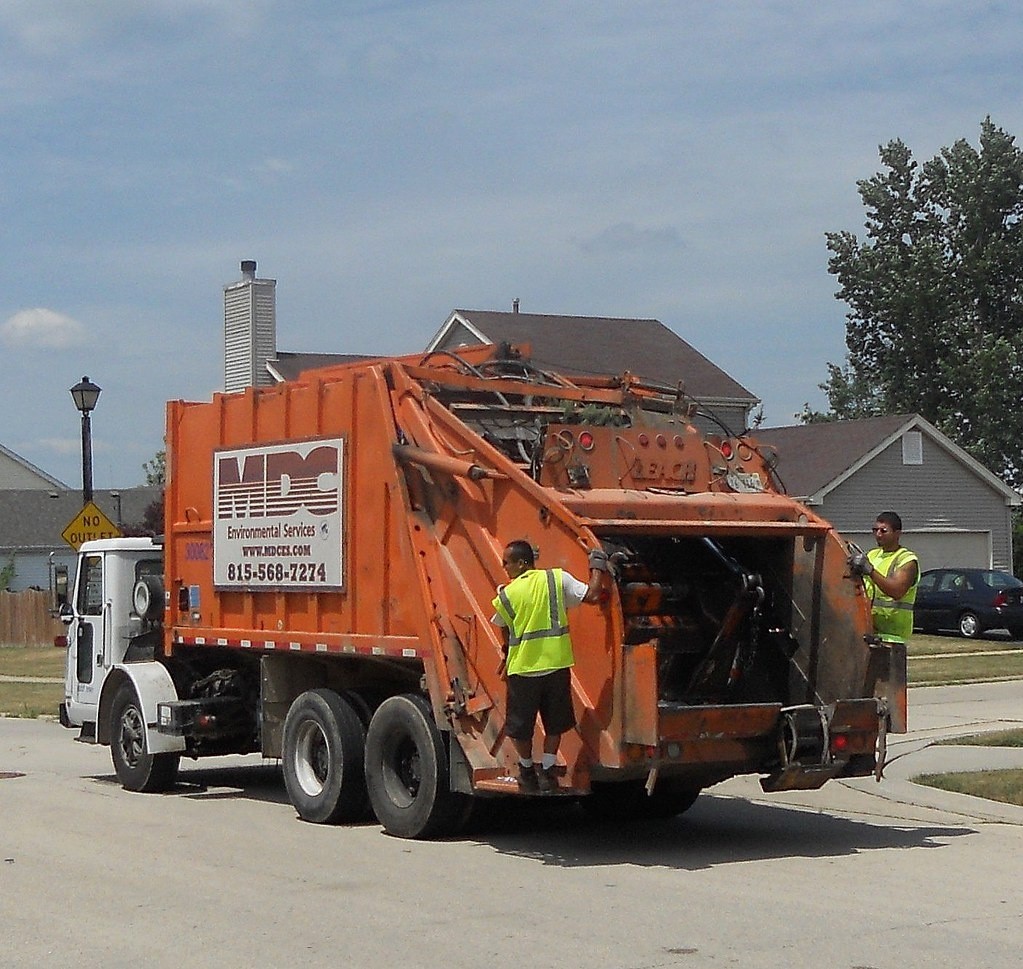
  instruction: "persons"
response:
[845,511,920,643]
[489,540,608,791]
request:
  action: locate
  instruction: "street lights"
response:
[69,375,101,613]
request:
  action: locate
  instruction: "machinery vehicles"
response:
[48,343,907,839]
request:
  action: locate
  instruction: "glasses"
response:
[502,559,528,567]
[872,527,895,535]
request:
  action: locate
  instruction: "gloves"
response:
[848,553,874,575]
[588,550,607,573]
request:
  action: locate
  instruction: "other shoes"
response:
[538,763,558,794]
[517,762,540,792]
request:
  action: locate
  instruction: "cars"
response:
[914,568,1022,641]
